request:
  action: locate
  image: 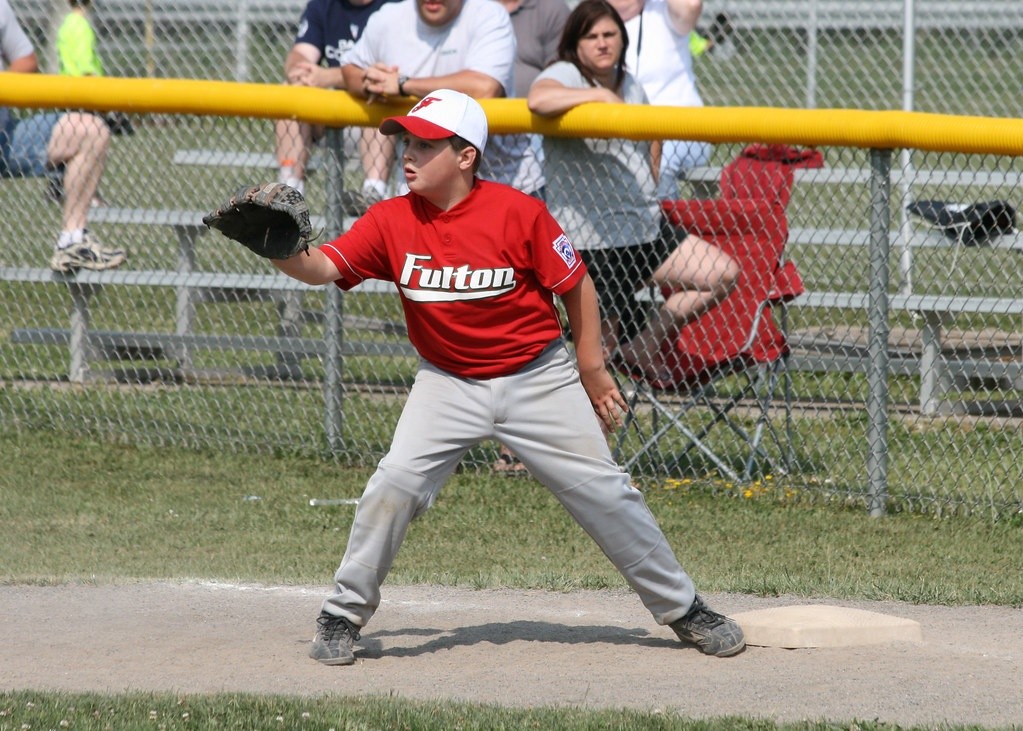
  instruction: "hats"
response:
[378,89,489,173]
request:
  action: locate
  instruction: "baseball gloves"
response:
[202,182,313,260]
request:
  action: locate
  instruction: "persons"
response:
[528,0,740,387]
[274,0,717,218]
[0,0,131,273]
[266,88,744,665]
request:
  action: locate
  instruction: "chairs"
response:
[607,201,801,484]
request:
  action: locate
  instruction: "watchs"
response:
[398,76,409,96]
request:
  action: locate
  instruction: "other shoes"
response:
[493,456,528,475]
[611,352,674,389]
[343,185,384,217]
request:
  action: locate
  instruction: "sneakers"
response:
[309,607,355,666]
[673,595,746,658]
[49,231,129,272]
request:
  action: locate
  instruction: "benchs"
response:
[1,148,1023,417]
